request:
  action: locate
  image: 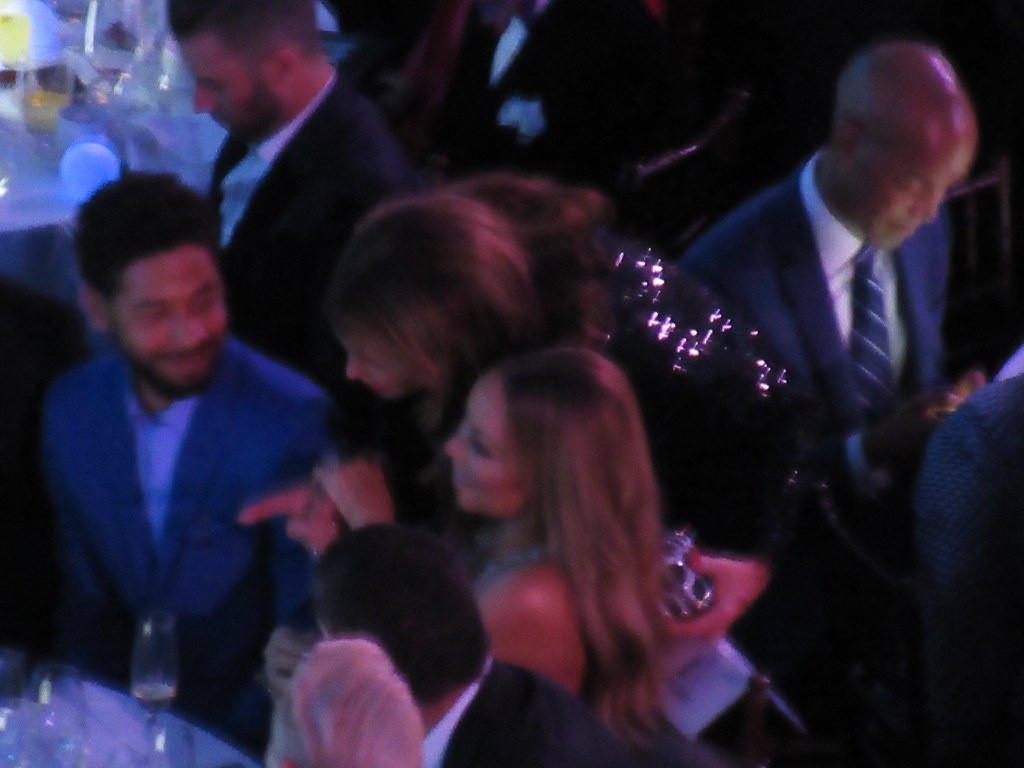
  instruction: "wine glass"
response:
[129,609,182,767]
[21,63,72,158]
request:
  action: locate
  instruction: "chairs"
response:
[945,149,1018,378]
[599,77,753,250]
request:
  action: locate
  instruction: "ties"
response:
[848,240,897,430]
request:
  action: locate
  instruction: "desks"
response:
[1,2,338,316]
[0,645,262,768]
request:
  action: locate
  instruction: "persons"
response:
[39,169,332,768]
[0,275,90,668]
[164,0,1024,768]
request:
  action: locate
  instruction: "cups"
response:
[0,651,89,768]
